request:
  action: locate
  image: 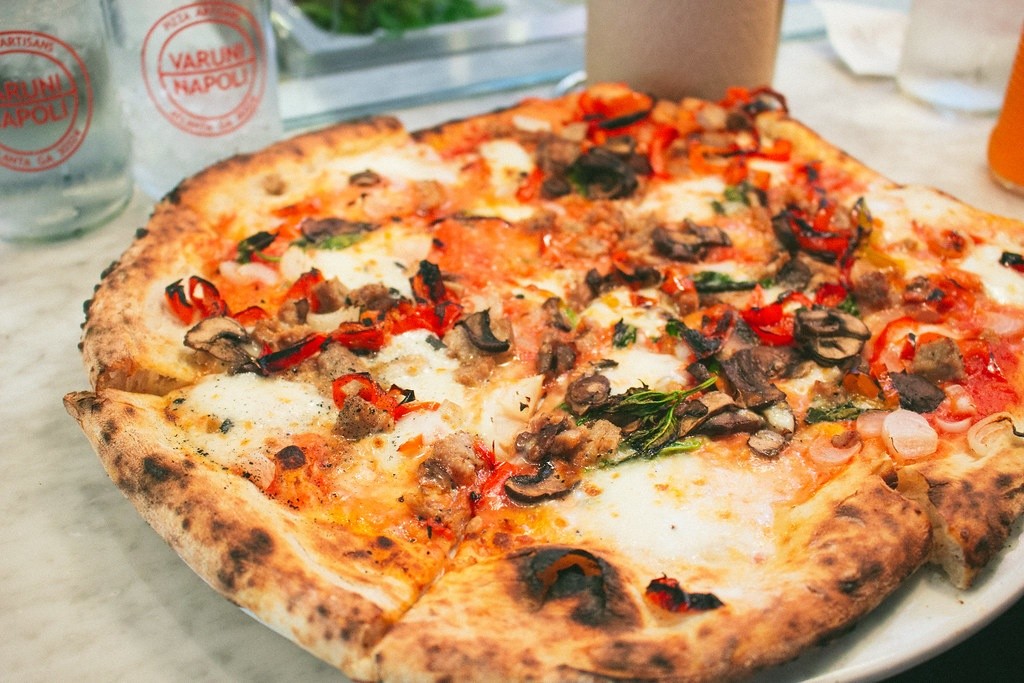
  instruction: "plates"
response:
[751,517,1023,683]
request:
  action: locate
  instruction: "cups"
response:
[587,0,784,107]
[988,20,1023,198]
[895,0,1024,111]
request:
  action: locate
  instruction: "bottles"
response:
[1,1,134,244]
[105,0,283,208]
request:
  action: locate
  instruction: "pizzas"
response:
[61,77,1024,681]
